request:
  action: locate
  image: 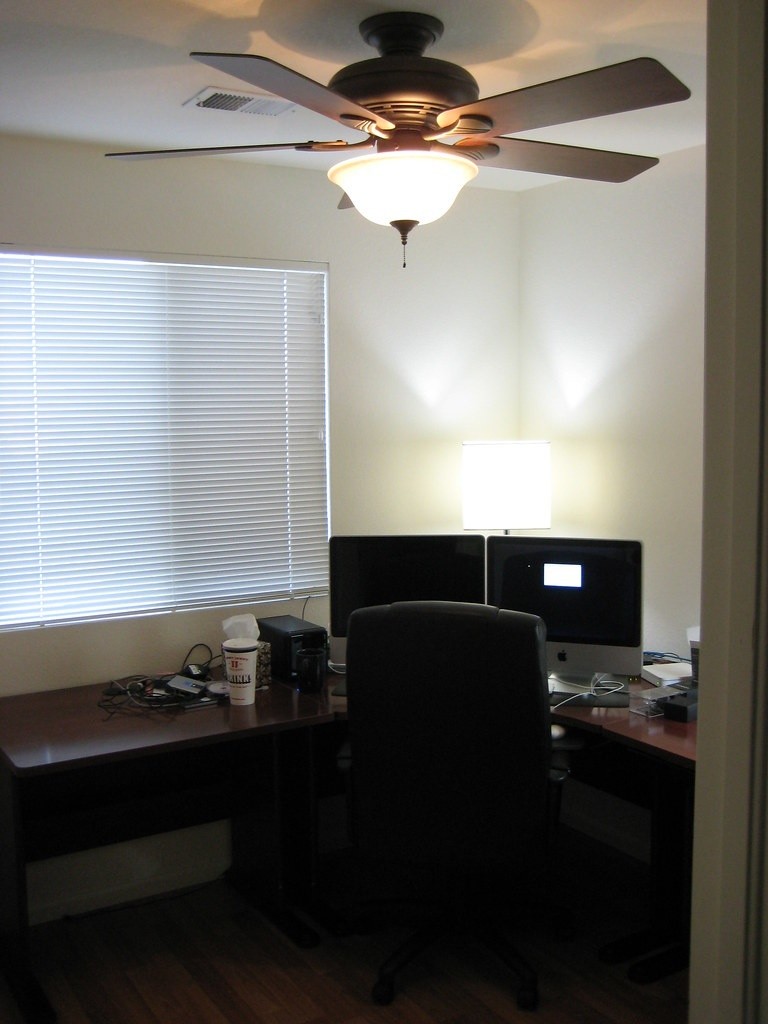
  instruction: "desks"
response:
[0,665,698,1020]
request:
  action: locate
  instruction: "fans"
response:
[105,11,692,210]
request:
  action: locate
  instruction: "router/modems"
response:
[642,663,692,687]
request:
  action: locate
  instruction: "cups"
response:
[222,638,258,704]
[296,649,327,693]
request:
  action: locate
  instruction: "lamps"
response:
[326,150,478,246]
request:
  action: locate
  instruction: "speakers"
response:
[256,614,329,692]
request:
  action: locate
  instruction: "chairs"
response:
[335,600,580,1012]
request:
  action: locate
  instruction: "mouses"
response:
[551,724,567,741]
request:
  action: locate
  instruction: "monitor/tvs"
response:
[329,534,486,637]
[487,536,643,707]
